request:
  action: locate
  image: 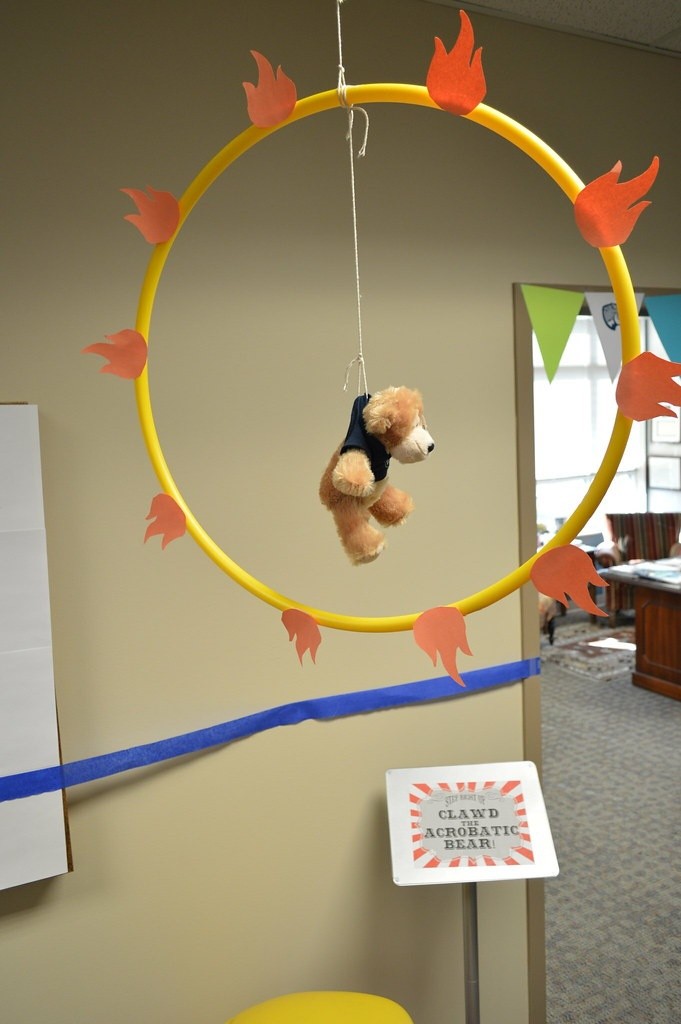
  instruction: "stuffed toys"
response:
[319,386,435,567]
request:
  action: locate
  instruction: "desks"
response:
[598,557,681,703]
[536,544,600,626]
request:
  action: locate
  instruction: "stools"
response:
[227,991,416,1024]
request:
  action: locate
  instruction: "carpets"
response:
[539,624,637,681]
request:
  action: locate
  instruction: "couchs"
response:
[595,511,681,629]
